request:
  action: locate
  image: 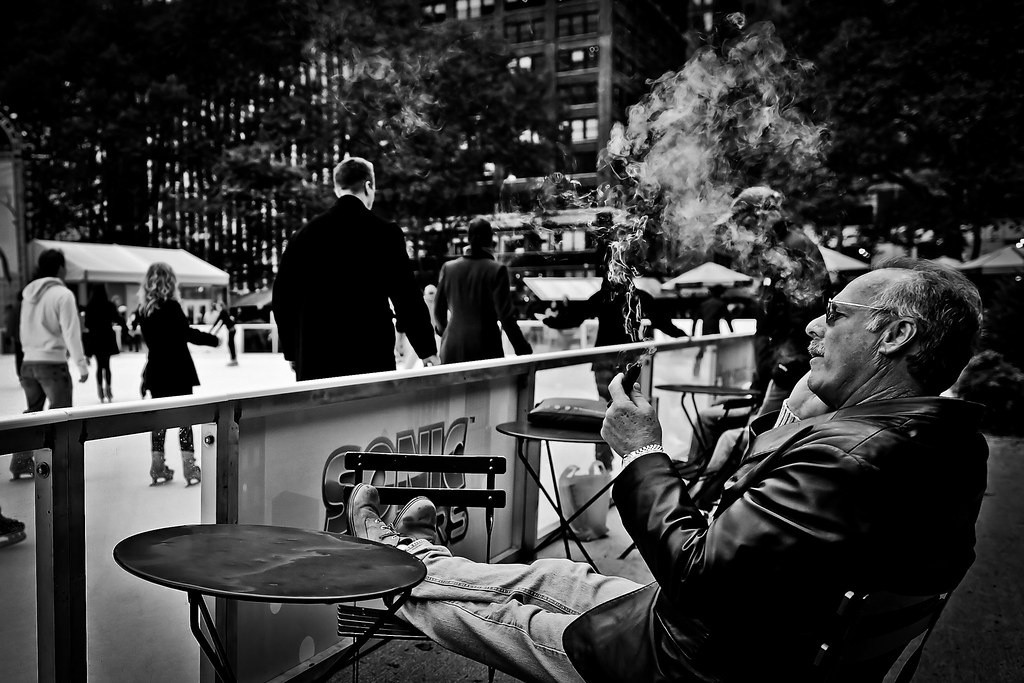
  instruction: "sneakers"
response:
[9,454,34,479]
[183,451,201,484]
[383,496,437,608]
[347,483,413,547]
[150,451,173,483]
[0,509,27,549]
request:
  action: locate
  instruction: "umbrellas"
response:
[661,243,1024,291]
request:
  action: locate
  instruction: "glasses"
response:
[826,298,883,323]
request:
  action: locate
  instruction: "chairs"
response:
[807,585,954,683]
[333,450,506,680]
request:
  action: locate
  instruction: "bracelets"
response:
[622,444,664,468]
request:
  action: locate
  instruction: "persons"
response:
[348,257,990,683]
[404,284,452,369]
[8,249,89,474]
[272,158,441,381]
[86,283,239,399]
[431,219,689,475]
[669,187,833,511]
[136,263,223,482]
[0,506,25,537]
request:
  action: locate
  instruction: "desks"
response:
[111,523,426,683]
[655,385,757,493]
[496,418,611,578]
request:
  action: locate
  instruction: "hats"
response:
[729,186,783,223]
[468,219,497,247]
